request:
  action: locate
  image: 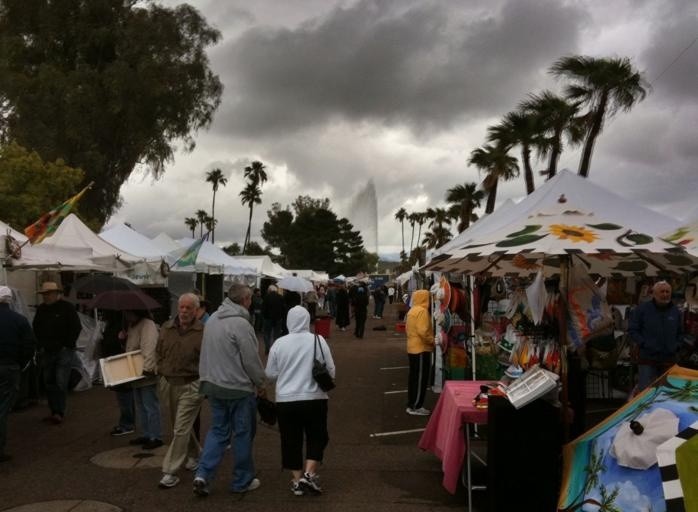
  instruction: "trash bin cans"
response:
[313,315,334,338]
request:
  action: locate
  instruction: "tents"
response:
[397,167,697,380]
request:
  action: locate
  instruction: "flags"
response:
[176,238,205,268]
[24,189,85,248]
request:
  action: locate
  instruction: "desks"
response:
[418,380,505,512]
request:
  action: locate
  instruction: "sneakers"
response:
[111,423,134,436]
[159,473,181,487]
[405,406,432,416]
[41,411,62,425]
[129,433,164,449]
[231,478,260,493]
[288,472,323,496]
[191,477,210,498]
[184,447,203,471]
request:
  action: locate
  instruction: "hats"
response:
[35,281,65,294]
[0,285,13,304]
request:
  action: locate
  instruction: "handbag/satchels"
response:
[312,358,336,392]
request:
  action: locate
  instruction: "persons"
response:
[264,305,337,497]
[628,280,683,392]
[250,279,395,354]
[31,282,82,424]
[405,289,435,415]
[0,285,38,463]
[92,292,210,489]
[192,284,265,497]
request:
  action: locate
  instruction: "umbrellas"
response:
[68,275,142,296]
[556,365,698,512]
[656,420,698,512]
